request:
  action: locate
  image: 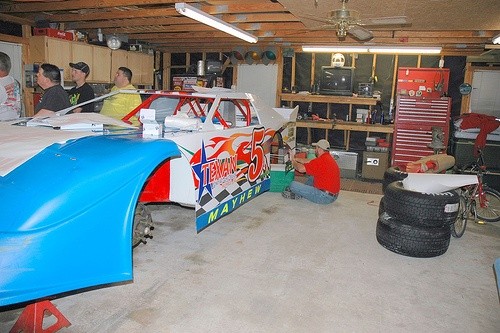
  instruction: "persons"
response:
[0,52,25,122]
[68,62,94,113]
[36,64,73,114]
[283,139,340,204]
[100,67,142,121]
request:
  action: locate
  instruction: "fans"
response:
[297,0,412,41]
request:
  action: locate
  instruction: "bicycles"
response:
[450,150,500,239]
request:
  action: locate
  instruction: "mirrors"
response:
[459,83,472,95]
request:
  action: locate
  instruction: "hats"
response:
[69,61,90,75]
[312,139,330,151]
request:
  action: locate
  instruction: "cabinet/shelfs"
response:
[29,36,154,85]
[279,94,394,151]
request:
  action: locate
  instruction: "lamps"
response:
[175,3,258,44]
[301,45,442,54]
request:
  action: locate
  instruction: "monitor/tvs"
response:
[319,66,355,96]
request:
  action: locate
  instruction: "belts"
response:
[320,189,339,197]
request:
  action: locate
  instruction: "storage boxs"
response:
[33,28,73,40]
[269,163,294,192]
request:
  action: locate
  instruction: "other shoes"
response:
[282,191,302,200]
[285,186,291,192]
